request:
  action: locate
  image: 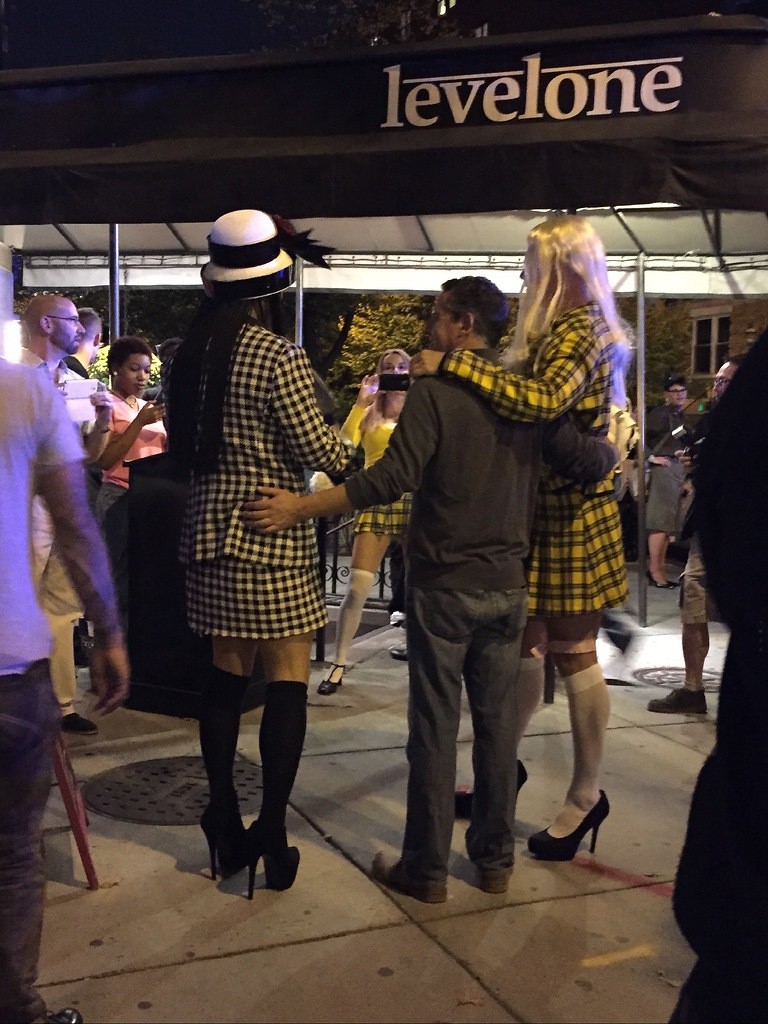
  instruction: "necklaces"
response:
[110,390,138,408]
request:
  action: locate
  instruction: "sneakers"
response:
[647,687,707,713]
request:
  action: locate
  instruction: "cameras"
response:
[672,424,707,467]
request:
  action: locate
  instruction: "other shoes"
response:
[474,866,514,892]
[390,649,408,661]
[49,1008,83,1024]
[60,713,97,734]
[373,850,448,903]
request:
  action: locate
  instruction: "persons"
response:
[165,211,365,898]
[0,295,183,1023]
[408,218,632,862]
[62,308,102,380]
[639,327,768,1024]
[20,294,112,735]
[240,275,619,903]
[316,351,412,696]
[94,337,168,650]
[389,540,406,625]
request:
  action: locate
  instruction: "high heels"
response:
[454,760,528,819]
[317,663,346,695]
[646,570,681,589]
[527,789,609,861]
[200,802,251,881]
[245,821,300,900]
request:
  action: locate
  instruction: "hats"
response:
[200,210,335,301]
[664,376,685,391]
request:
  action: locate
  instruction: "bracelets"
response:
[94,419,111,433]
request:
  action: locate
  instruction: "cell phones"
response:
[63,378,98,399]
[378,374,410,391]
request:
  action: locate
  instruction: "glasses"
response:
[46,316,79,327]
[667,388,687,393]
